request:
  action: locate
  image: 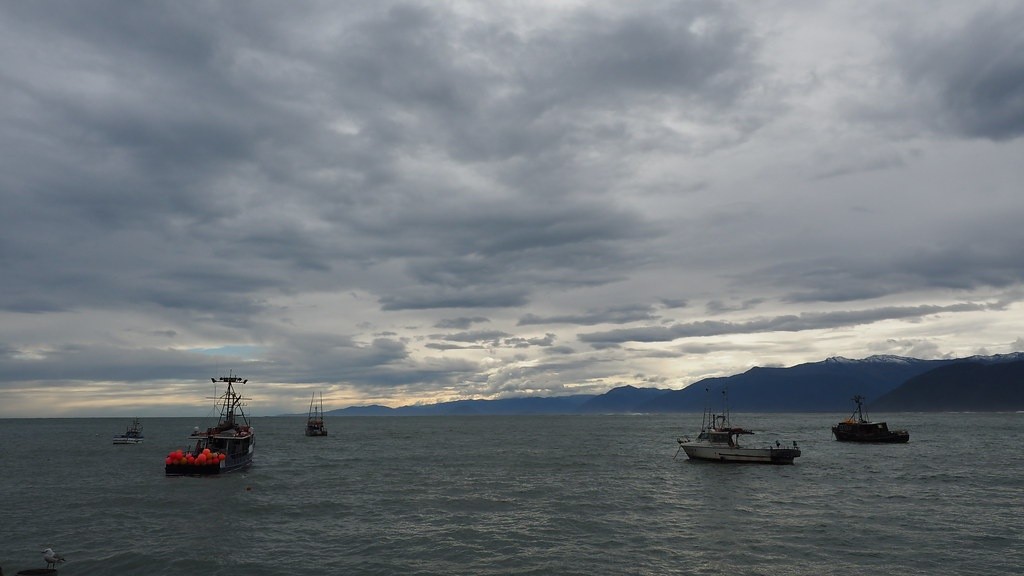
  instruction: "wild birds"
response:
[41,548,66,570]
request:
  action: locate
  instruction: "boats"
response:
[828,392,911,445]
[111,417,145,445]
[162,365,257,476]
[675,387,801,462]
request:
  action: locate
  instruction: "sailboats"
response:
[303,391,329,437]
